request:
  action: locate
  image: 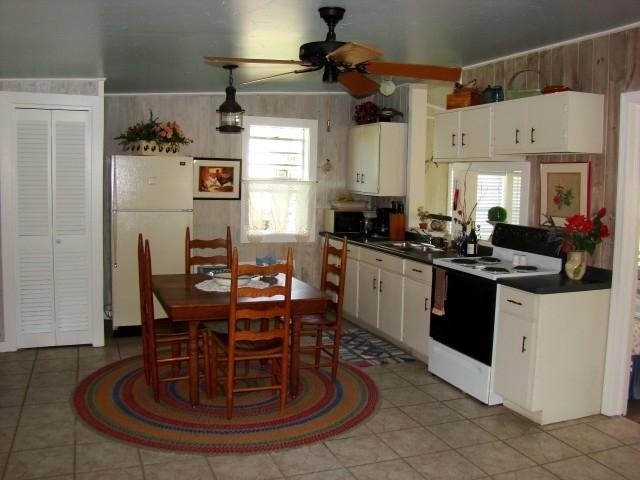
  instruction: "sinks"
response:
[384,241,424,248]
[395,246,442,253]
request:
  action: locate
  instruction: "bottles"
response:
[468,228,478,255]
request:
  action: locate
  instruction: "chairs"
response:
[138,232,204,367]
[209,247,293,419]
[273,231,347,397]
[186,225,236,354]
[144,238,211,401]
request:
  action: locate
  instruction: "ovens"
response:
[428,264,501,407]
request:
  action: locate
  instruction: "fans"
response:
[203,6,462,101]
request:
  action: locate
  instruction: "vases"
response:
[456,227,468,256]
[566,249,587,279]
[125,140,178,155]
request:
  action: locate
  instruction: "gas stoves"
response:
[433,255,560,281]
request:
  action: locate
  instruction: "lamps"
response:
[379,74,395,96]
[321,62,339,84]
[215,64,248,132]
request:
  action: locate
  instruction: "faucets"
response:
[422,229,432,244]
[410,228,421,243]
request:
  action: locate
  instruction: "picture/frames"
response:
[192,158,242,200]
[540,161,591,228]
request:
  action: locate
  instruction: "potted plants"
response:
[417,207,429,229]
[486,206,508,242]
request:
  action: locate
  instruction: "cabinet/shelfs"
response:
[346,122,406,198]
[320,236,359,327]
[493,90,606,159]
[432,104,493,163]
[0,77,106,352]
[401,257,432,368]
[494,282,543,427]
[355,245,403,353]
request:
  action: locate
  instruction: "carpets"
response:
[72,342,379,456]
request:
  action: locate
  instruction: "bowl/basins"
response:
[208,270,256,286]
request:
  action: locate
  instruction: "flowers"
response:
[452,164,480,225]
[542,207,609,256]
[351,102,379,125]
[114,118,194,152]
